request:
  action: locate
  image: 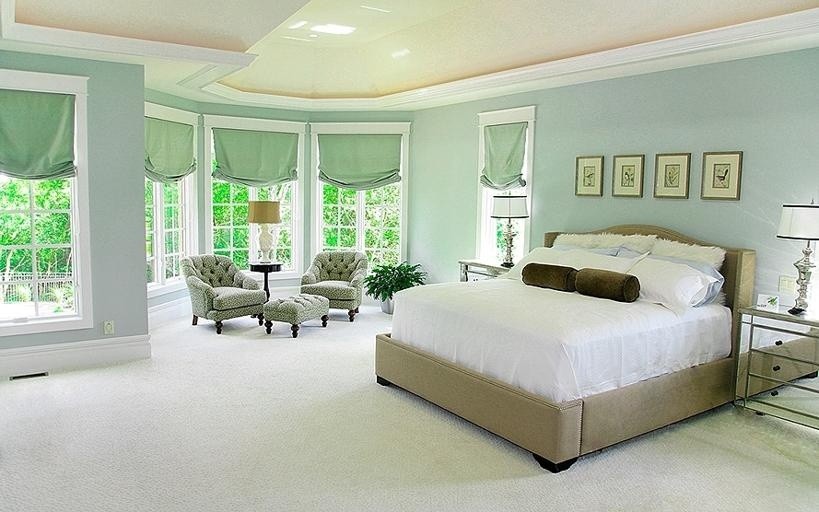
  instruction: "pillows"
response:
[627,259,714,311]
[616,245,725,307]
[506,247,627,280]
[521,263,576,292]
[551,244,618,258]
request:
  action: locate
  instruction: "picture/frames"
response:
[612,154,644,197]
[574,155,605,197]
[701,151,743,202]
[653,153,691,199]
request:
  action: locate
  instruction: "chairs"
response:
[301,251,368,322]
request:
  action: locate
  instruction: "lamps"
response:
[491,195,530,266]
[247,200,283,264]
[775,198,819,316]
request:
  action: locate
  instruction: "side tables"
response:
[250,264,281,321]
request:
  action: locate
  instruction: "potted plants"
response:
[364,260,428,314]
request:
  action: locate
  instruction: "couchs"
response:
[262,292,330,339]
[181,254,267,335]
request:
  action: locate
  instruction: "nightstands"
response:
[732,304,819,431]
[457,257,517,281]
[575,267,640,302]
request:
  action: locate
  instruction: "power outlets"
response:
[102,320,116,336]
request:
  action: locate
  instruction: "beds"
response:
[375,223,758,472]
